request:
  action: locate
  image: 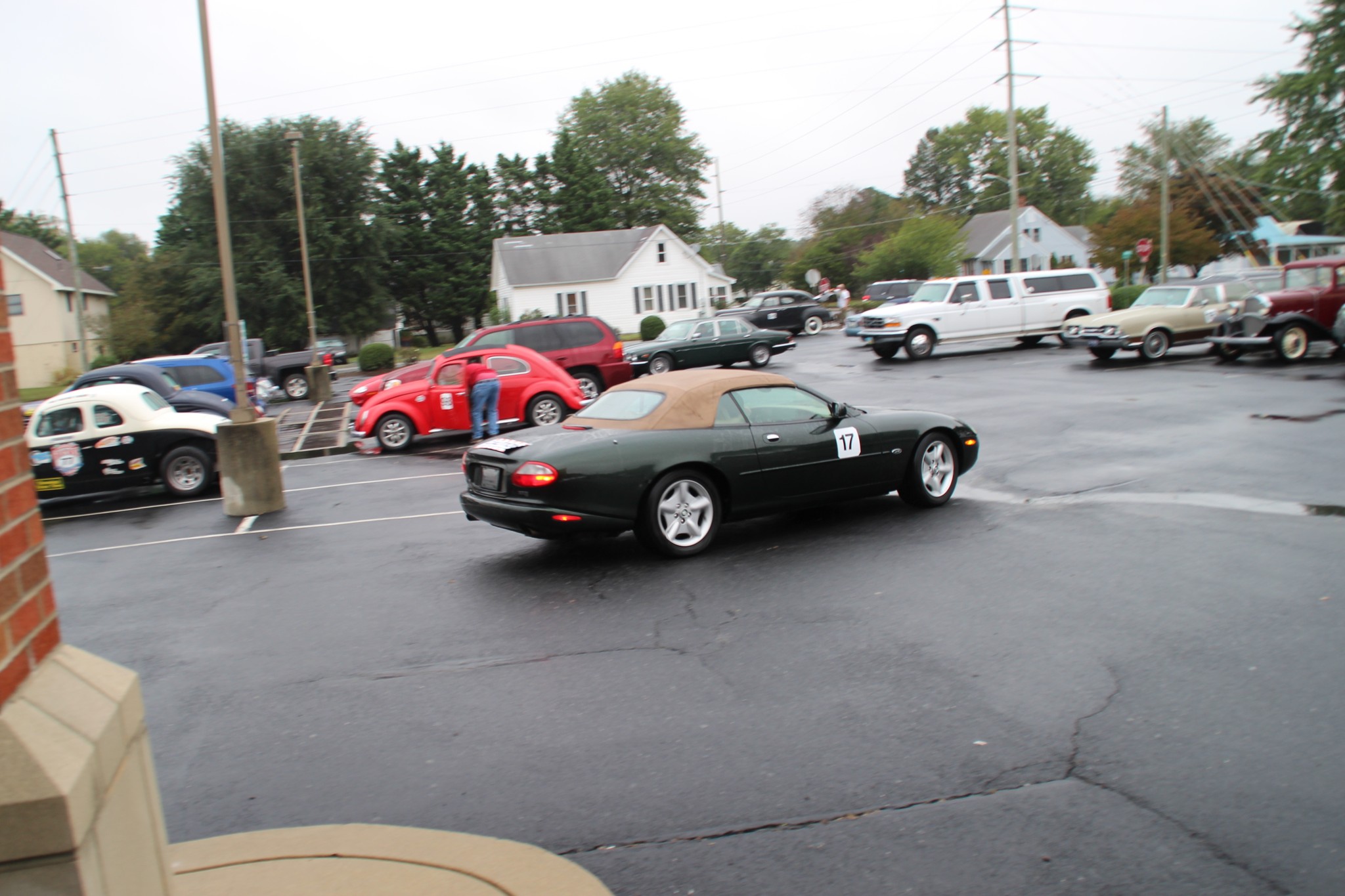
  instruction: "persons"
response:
[461,362,500,446]
[834,283,850,332]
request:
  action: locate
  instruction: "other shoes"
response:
[487,434,498,439]
[469,437,483,444]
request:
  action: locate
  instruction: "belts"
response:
[474,378,498,385]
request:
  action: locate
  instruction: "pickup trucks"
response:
[121,336,339,415]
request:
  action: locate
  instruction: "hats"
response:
[837,283,846,289]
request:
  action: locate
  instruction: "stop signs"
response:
[817,277,831,294]
[1136,238,1154,258]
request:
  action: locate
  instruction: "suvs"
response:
[348,313,635,408]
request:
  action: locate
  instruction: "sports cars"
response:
[27,363,238,421]
[24,383,232,505]
[458,366,978,562]
[347,341,599,456]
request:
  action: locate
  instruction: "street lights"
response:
[282,126,324,365]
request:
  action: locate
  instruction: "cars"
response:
[304,335,349,365]
[714,287,839,335]
[844,278,925,345]
[1059,252,1345,369]
[621,314,799,377]
[844,267,1114,361]
